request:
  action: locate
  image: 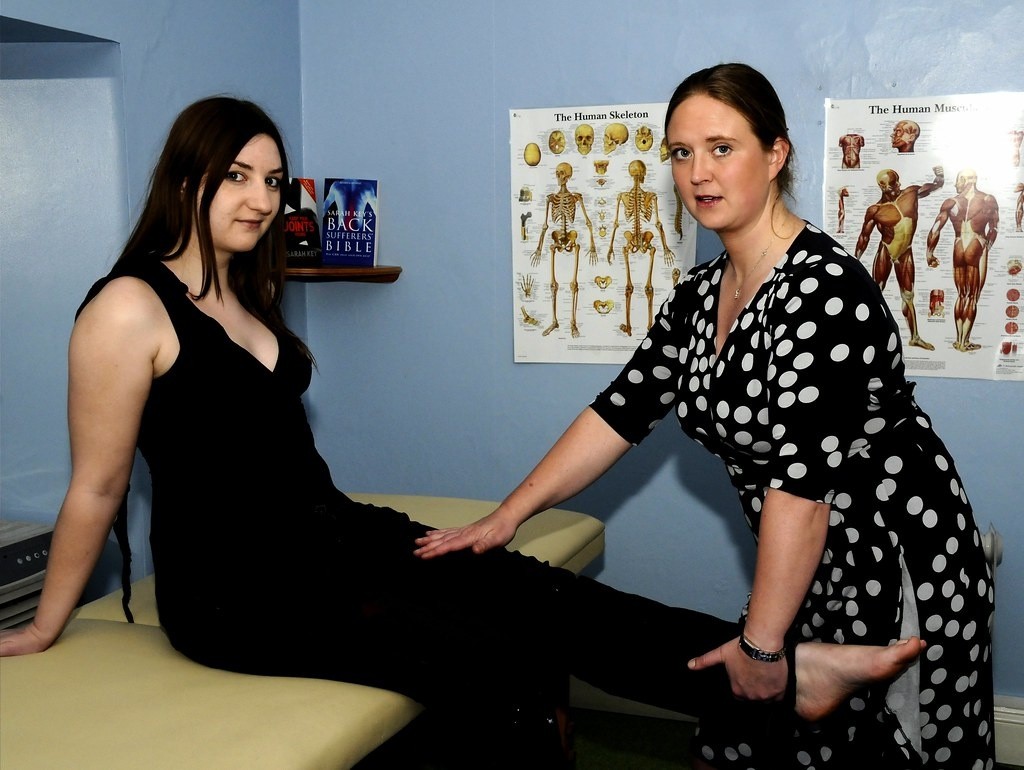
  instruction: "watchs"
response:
[739,633,785,663]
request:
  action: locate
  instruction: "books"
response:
[282,177,322,268]
[322,177,380,267]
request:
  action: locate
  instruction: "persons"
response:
[413,64,998,770]
[0,97,927,770]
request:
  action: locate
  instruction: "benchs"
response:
[0,490,604,770]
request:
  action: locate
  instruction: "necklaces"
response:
[734,220,786,299]
